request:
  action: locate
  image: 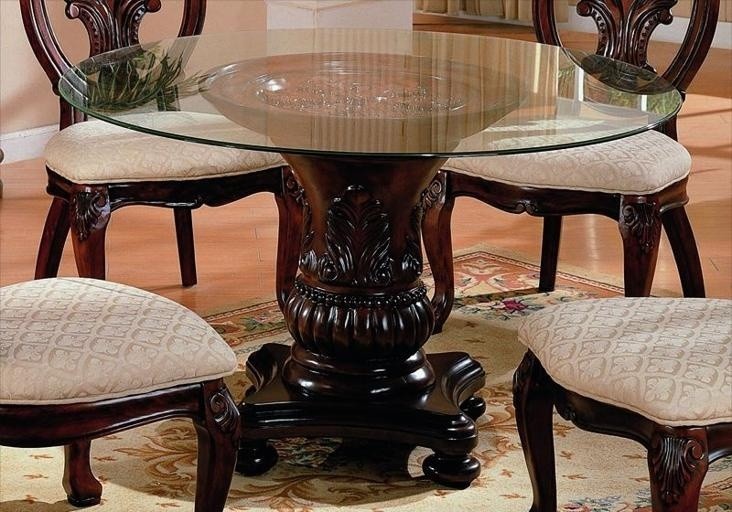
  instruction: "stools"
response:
[1,277,244,512]
[515,295,732,511]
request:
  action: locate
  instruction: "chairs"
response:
[17,0,290,287]
[409,0,723,336]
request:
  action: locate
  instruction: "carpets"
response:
[1,243,731,510]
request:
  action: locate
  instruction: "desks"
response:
[56,26,683,491]
